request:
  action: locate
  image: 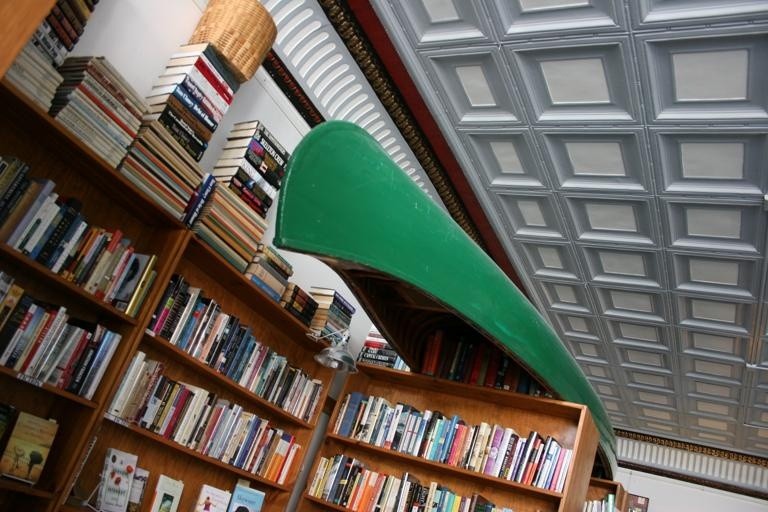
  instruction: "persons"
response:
[197,496,216,511]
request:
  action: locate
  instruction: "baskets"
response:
[188,0,278,84]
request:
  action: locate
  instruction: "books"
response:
[95,448,139,511]
[308,283,650,512]
[147,472,185,512]
[189,483,232,512]
[227,483,266,512]
[126,466,151,511]
[0,1,325,490]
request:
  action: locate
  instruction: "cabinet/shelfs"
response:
[48,227,342,511]
[0,76,187,511]
